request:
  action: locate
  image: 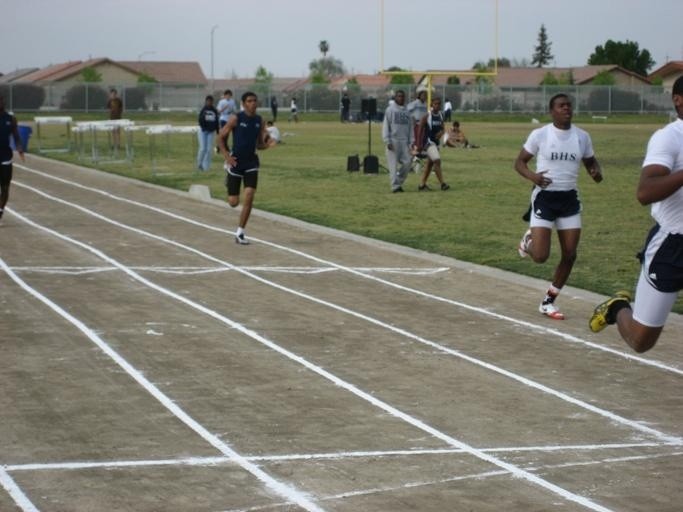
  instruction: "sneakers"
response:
[519,229,532,257]
[589,291,631,332]
[539,302,564,319]
[441,183,449,189]
[419,185,429,191]
[236,233,248,244]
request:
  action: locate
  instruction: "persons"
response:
[380,90,479,193]
[216,92,272,244]
[106,89,122,148]
[587,76,682,354]
[270,96,278,122]
[0,90,24,220]
[340,93,352,123]
[217,90,237,154]
[513,94,603,321]
[261,120,280,146]
[287,97,298,124]
[195,95,218,172]
